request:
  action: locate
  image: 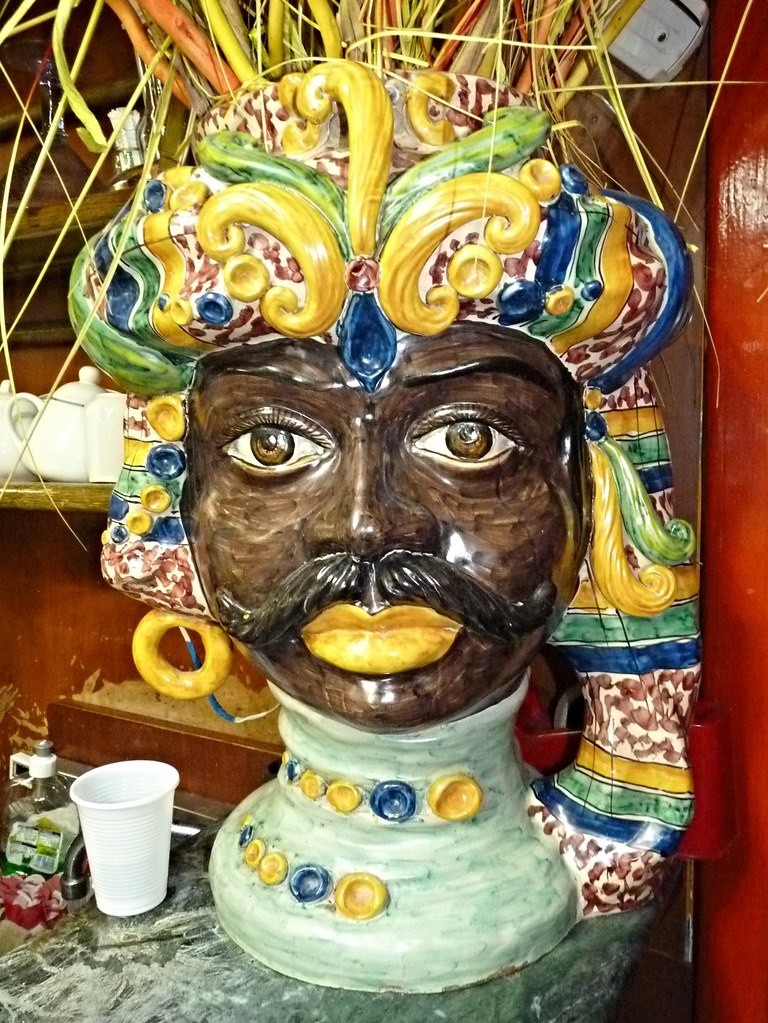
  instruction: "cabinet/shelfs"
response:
[0,0,703,636]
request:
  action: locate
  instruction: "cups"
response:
[68,759,180,920]
[84,392,127,483]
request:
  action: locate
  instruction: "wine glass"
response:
[0,40,106,213]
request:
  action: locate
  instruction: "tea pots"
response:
[5,365,111,481]
[0,379,41,482]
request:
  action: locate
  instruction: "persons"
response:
[69,74,694,728]
